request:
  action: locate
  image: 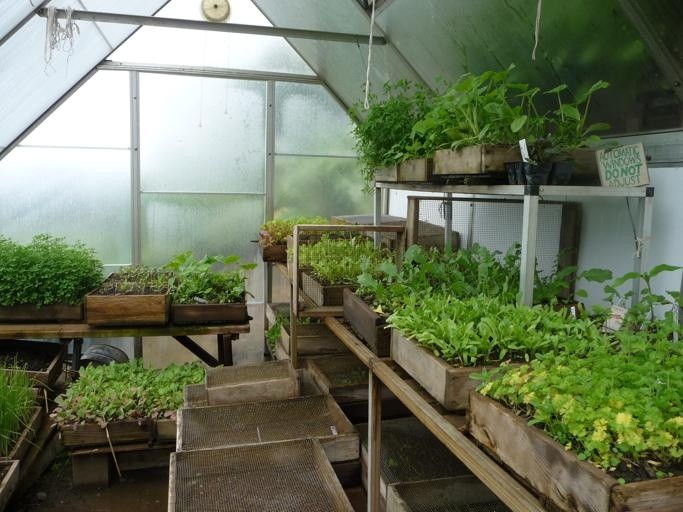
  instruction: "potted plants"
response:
[0,231,103,325]
[166,251,260,324]
[0,351,43,459]
[49,358,207,446]
[348,62,611,185]
[85,263,178,325]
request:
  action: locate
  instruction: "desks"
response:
[0,319,249,368]
